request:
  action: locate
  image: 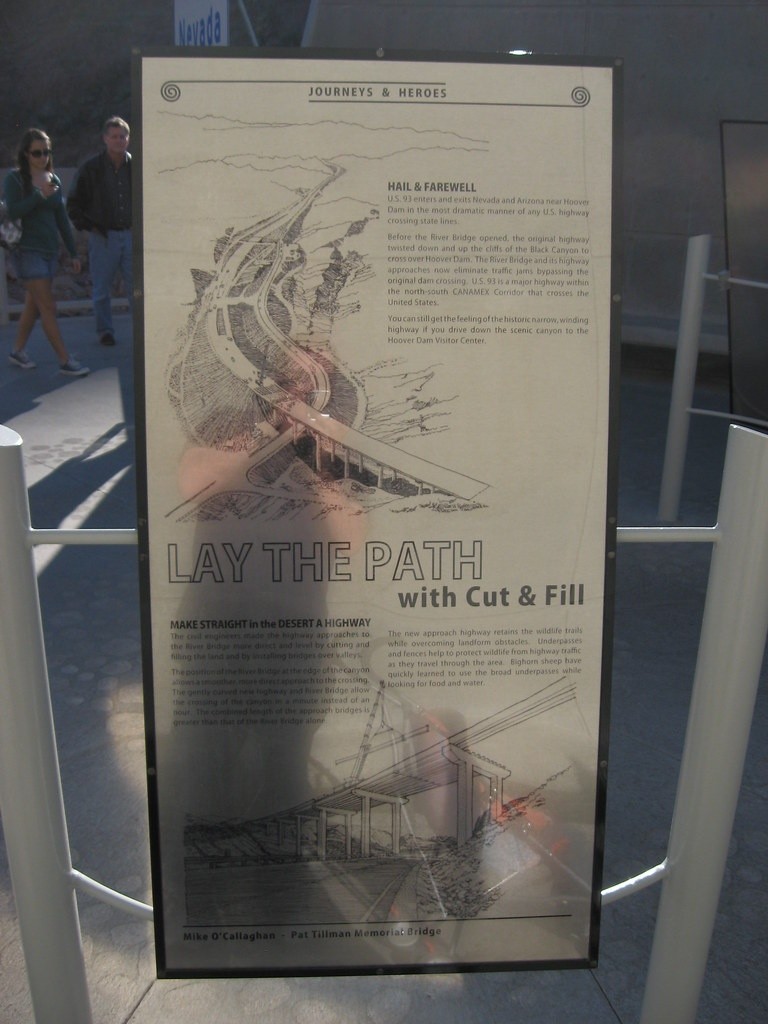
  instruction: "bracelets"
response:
[40,191,48,201]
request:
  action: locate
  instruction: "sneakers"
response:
[59,353,90,376]
[8,350,36,369]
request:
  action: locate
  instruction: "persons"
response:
[0,128,90,375]
[66,117,132,346]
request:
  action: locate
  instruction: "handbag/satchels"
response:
[0,168,24,251]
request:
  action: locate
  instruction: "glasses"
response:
[26,148,52,158]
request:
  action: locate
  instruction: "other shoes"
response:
[100,333,115,346]
